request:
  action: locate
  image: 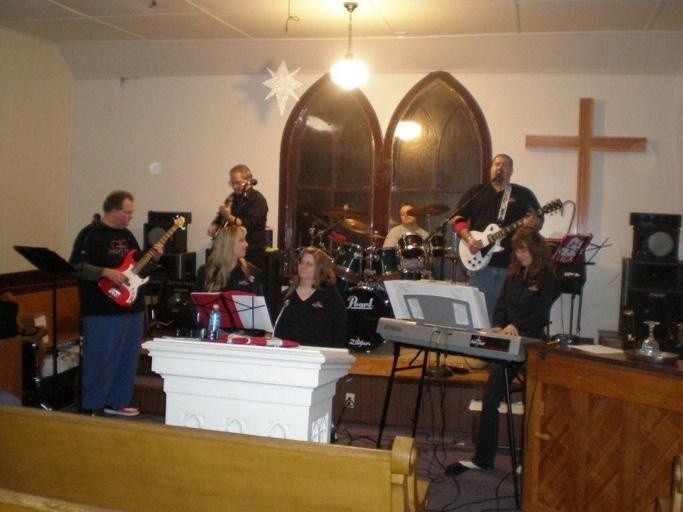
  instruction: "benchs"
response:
[1,402,433,510]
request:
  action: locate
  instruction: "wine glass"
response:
[642,321,660,356]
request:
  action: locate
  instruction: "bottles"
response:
[207,304,222,342]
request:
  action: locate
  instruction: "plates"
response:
[626,350,678,364]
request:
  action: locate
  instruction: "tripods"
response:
[44,278,70,410]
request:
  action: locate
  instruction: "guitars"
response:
[98,214,188,309]
[459,198,565,271]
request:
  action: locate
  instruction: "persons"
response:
[459,227,558,469]
[208,165,268,265]
[266,248,349,351]
[70,192,163,417]
[448,153,544,330]
[348,201,434,351]
[189,225,266,338]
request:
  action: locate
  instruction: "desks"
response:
[522,336,682,510]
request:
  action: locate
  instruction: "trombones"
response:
[212,177,257,239]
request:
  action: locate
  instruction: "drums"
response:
[396,231,424,259]
[400,270,423,281]
[332,241,362,284]
[431,235,446,257]
[375,247,399,276]
[362,246,379,276]
[333,264,359,298]
[343,281,393,353]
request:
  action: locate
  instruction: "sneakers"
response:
[459,460,485,470]
[103,407,139,416]
[516,465,522,475]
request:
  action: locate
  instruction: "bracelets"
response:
[465,236,472,243]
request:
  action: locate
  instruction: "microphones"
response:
[268,299,290,340]
[299,209,332,230]
[237,179,258,189]
[487,169,504,189]
[82,214,101,243]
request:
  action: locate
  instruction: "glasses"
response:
[228,181,244,186]
[298,261,316,267]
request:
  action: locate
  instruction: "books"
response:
[191,289,275,334]
[384,278,491,335]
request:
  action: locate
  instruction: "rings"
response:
[474,244,477,250]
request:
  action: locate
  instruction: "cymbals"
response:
[340,217,371,233]
[319,208,369,221]
[298,211,328,228]
[406,203,450,217]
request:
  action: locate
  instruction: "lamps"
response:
[395,120,424,146]
[331,3,370,92]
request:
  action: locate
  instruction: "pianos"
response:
[375,313,545,365]
